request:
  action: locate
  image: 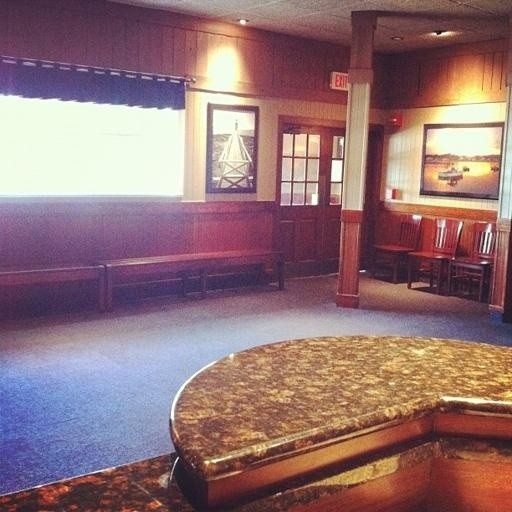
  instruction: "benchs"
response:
[2,250,287,314]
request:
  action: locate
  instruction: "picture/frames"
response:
[418,123,504,199]
[205,101,259,193]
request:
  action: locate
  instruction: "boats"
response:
[438,168,463,180]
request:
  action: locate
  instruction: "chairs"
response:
[370,213,496,302]
